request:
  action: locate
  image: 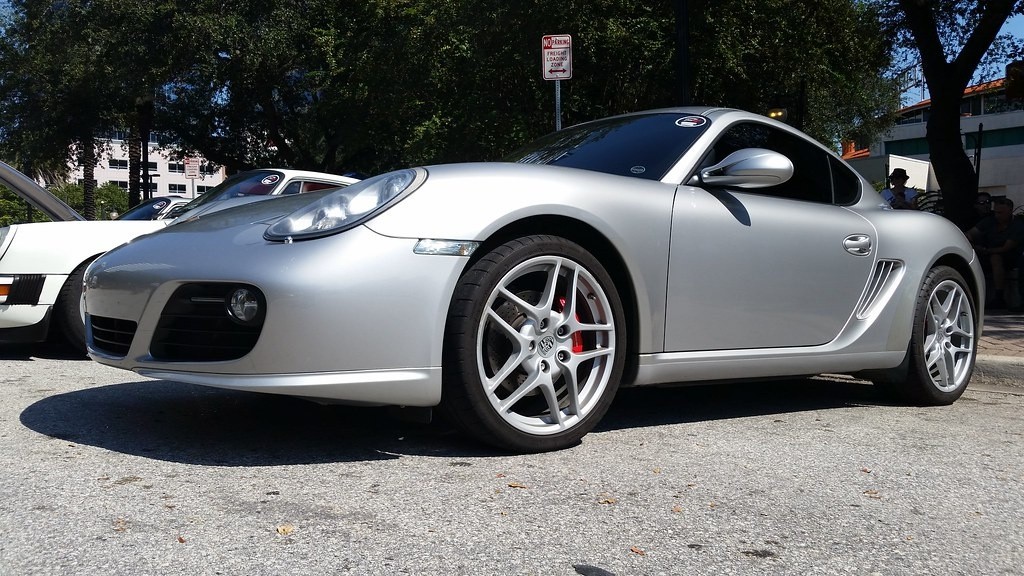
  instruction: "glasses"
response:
[976,198,992,206]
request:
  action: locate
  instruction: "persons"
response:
[959,192,1024,313]
[881,168,919,211]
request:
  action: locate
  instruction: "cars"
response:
[0,160,195,221]
[0,168,363,357]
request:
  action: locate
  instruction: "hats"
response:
[888,168,910,182]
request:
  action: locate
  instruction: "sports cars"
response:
[81,107,985,453]
